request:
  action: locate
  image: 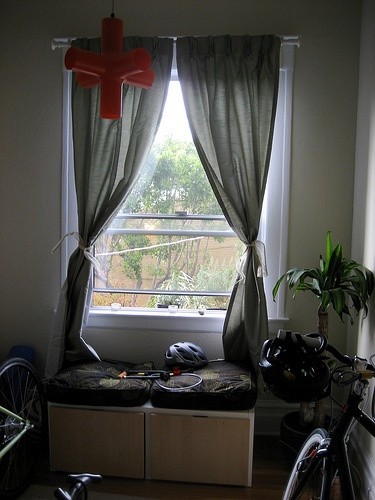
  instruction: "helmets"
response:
[164,340,209,371]
[259,337,332,404]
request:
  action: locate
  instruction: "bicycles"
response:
[277,330,375,500]
[0,357,102,500]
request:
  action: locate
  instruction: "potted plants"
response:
[274,229,374,466]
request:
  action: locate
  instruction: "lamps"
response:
[63,1,154,119]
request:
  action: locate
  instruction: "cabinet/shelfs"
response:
[46,397,255,487]
